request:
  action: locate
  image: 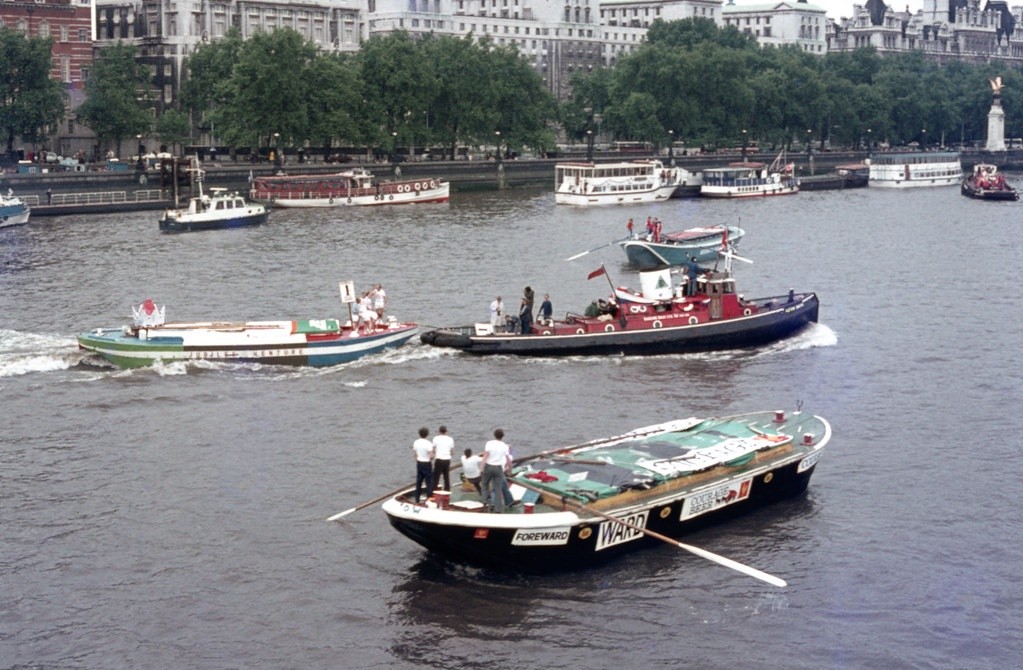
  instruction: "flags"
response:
[723,225,729,250]
[588,266,604,280]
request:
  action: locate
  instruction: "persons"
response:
[491,286,552,335]
[350,284,387,338]
[432,425,454,493]
[461,428,521,514]
[412,427,436,505]
[626,214,662,244]
[687,256,711,297]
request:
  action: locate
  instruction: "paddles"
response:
[505,476,788,588]
[325,452,484,522]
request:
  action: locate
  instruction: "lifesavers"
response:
[375,196,378,200]
[767,178,771,184]
[397,185,404,192]
[986,167,992,173]
[430,181,435,189]
[422,182,428,190]
[404,185,411,192]
[270,197,275,204]
[415,183,420,190]
[348,199,351,203]
[786,165,792,171]
[389,196,393,200]
[329,199,333,204]
[676,303,694,312]
[381,196,384,200]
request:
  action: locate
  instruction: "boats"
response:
[419,265,820,355]
[0,196,32,228]
[249,167,450,207]
[619,220,746,271]
[553,152,963,205]
[76,317,419,368]
[382,409,834,576]
[159,187,270,230]
[961,162,1019,201]
